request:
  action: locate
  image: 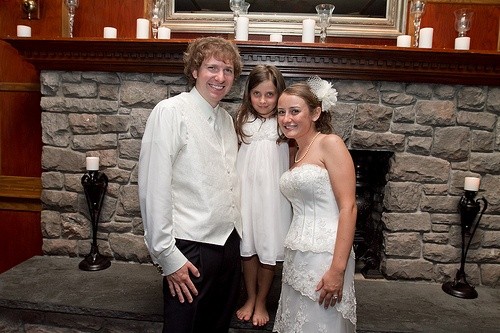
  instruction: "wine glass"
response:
[454,8,474,36]
[315,4,335,43]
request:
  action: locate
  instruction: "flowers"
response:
[316,80,338,112]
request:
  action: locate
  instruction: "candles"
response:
[464,175,481,193]
[270,33,282,41]
[454,34,469,50]
[299,19,317,42]
[135,17,150,39]
[16,25,34,38]
[233,17,249,40]
[85,155,100,173]
[417,27,434,47]
[157,25,171,39]
[102,26,118,40]
[396,32,413,47]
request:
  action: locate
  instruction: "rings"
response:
[332,296,338,299]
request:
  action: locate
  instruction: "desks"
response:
[0,256,500,333]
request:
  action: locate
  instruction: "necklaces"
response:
[295,132,321,163]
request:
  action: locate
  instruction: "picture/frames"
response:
[142,0,409,39]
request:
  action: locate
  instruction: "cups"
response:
[230,0,250,40]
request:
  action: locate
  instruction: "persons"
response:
[234,65,298,326]
[273,75,357,333]
[138,36,242,333]
